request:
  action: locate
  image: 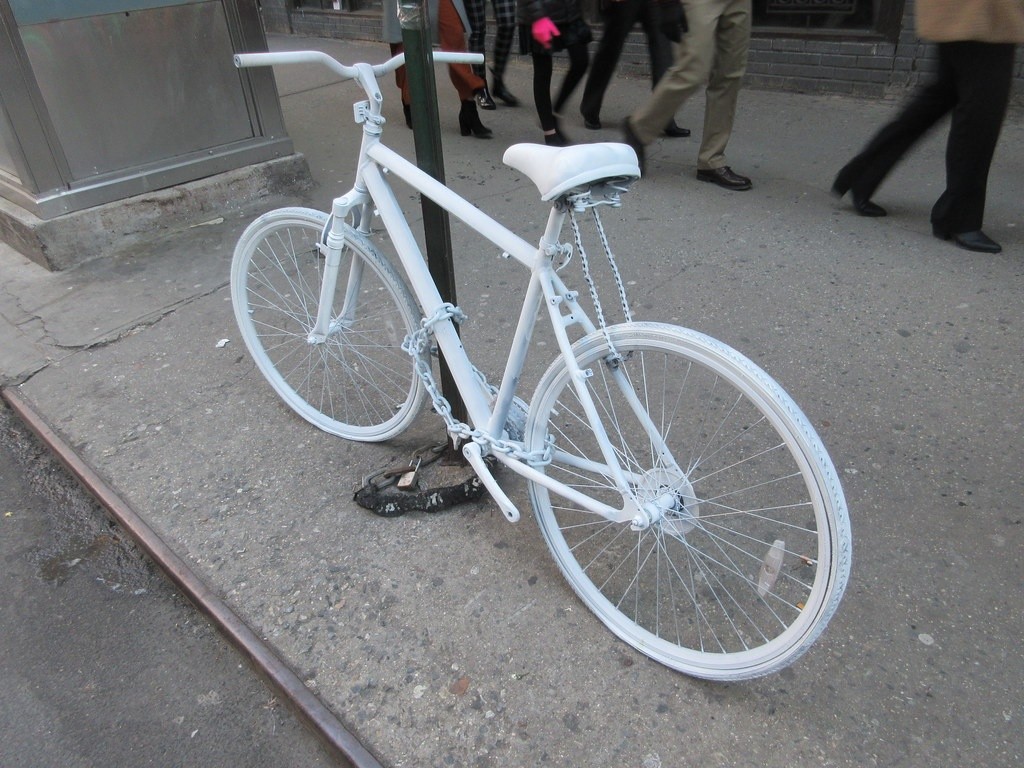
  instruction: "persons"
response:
[580,0,691,136]
[382,0,494,138]
[832,0,1024,253]
[517,0,595,147]
[622,0,753,190]
[461,0,517,111]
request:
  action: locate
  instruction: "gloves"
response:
[660,0,688,43]
[531,17,560,50]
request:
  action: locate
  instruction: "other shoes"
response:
[543,133,566,147]
[538,114,568,142]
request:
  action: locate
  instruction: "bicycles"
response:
[229,51,852,682]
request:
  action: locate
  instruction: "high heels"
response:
[831,171,887,218]
[459,100,493,137]
[402,100,413,130]
[933,224,1002,253]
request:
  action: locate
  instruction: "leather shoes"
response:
[584,118,601,130]
[696,166,752,190]
[492,87,519,105]
[477,87,496,110]
[664,120,691,137]
[621,116,645,178]
